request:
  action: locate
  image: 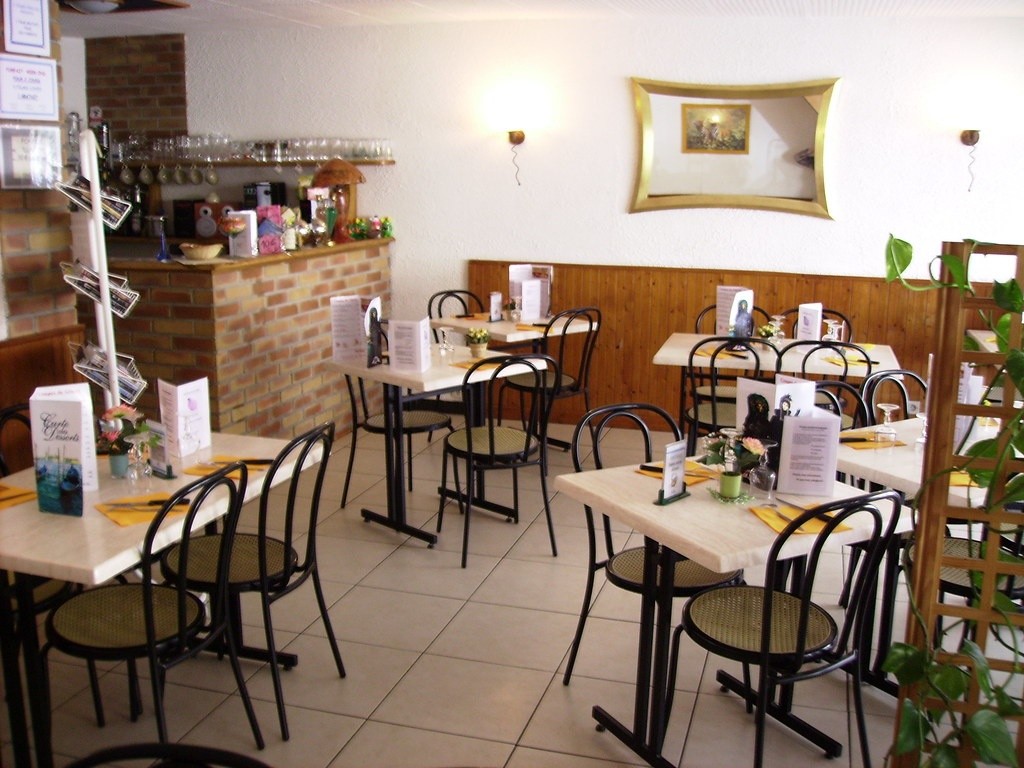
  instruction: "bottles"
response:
[314,194,337,242]
[101,173,144,237]
[368,214,392,238]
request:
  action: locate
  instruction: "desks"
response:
[0,432,332,768]
[430,309,598,449]
[820,416,1024,704]
[966,330,1007,374]
[330,344,548,543]
[653,331,904,456]
[553,459,920,768]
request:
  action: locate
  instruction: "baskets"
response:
[178,242,224,260]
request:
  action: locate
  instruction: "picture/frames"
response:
[680,104,751,154]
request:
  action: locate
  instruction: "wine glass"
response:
[115,130,394,162]
[719,427,743,473]
[438,327,454,362]
[821,319,843,352]
[768,316,785,352]
[916,413,930,459]
[873,404,900,455]
[749,440,778,498]
[509,296,522,322]
[122,432,154,493]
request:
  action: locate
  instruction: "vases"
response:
[718,472,741,498]
[470,343,487,360]
[107,455,129,482]
[762,336,772,352]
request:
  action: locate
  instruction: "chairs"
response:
[0,295,1024,768]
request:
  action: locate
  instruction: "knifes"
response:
[639,464,716,479]
[845,358,880,365]
[211,460,278,465]
[104,499,191,505]
[776,497,835,524]
[718,350,748,360]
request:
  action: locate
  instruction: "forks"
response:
[685,466,718,475]
[761,503,804,531]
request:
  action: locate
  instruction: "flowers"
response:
[755,324,775,338]
[465,327,488,345]
[703,432,765,476]
[94,404,161,464]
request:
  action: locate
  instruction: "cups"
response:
[146,215,166,238]
